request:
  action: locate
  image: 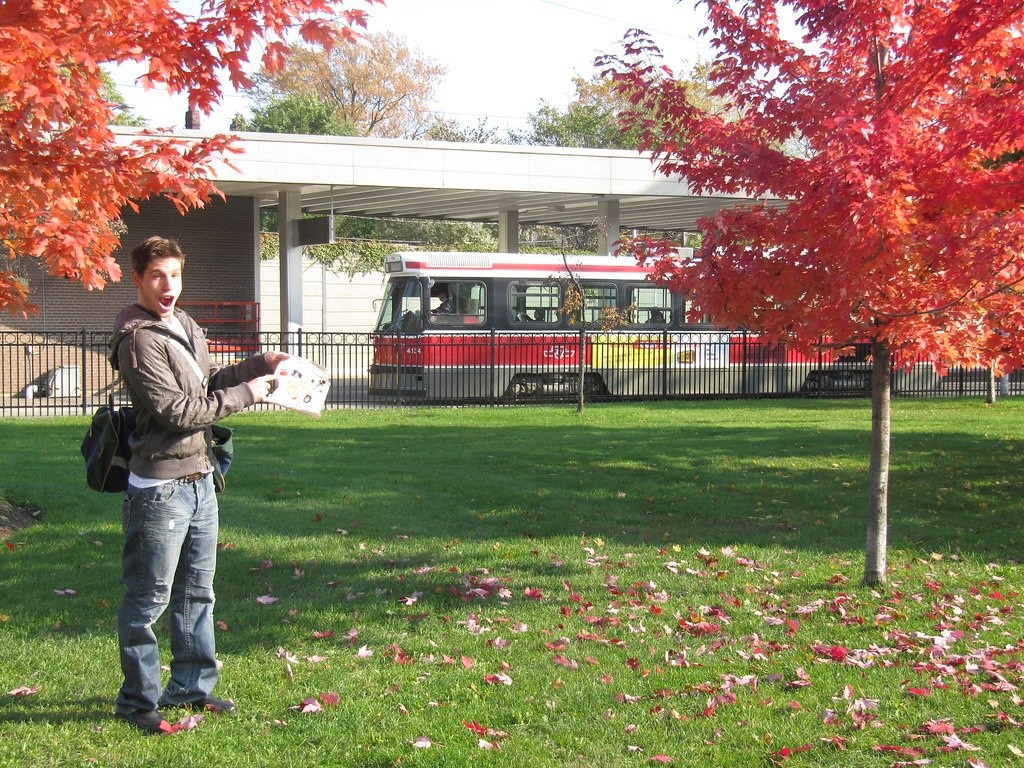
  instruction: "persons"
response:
[432,288,455,313]
[108,237,291,733]
[645,307,666,323]
[520,307,545,321]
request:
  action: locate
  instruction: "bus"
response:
[368,251,944,403]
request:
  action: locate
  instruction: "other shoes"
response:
[189,696,233,713]
[115,709,168,734]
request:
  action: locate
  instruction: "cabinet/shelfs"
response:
[178,301,260,353]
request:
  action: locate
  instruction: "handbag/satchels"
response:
[81,406,233,494]
